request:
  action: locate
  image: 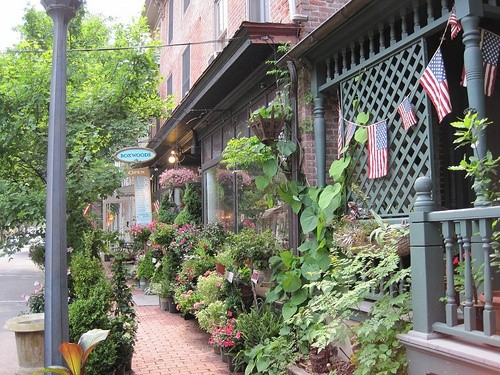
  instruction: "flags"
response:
[449,8,462,39]
[344,123,357,148]
[367,120,388,179]
[337,117,343,158]
[399,98,417,130]
[461,29,500,97]
[153,201,160,215]
[419,49,452,124]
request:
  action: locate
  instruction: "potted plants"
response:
[224,308,282,375]
[212,229,285,287]
[136,258,156,291]
[246,103,293,146]
[161,251,180,313]
[101,245,110,261]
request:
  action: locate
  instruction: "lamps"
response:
[168,149,178,166]
[149,174,154,181]
[153,164,160,172]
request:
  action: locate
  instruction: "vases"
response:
[220,347,232,362]
[184,312,195,320]
[448,110,500,335]
[214,347,221,354]
[460,300,484,331]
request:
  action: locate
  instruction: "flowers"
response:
[158,169,197,189]
[22,220,241,348]
[439,253,484,305]
